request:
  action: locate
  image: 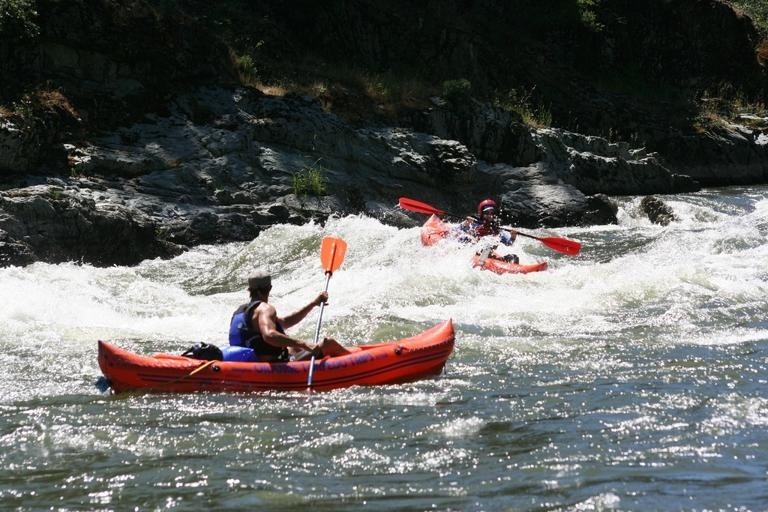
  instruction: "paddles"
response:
[398,195,581,257]
[307,237,346,393]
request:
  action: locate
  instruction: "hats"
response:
[248,270,271,289]
[483,206,495,211]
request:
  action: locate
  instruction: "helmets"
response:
[478,199,496,216]
[191,343,223,361]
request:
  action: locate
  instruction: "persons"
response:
[456,198,520,267]
[229,269,329,361]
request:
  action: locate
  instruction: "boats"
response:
[97,317,454,391]
[423,212,549,273]
